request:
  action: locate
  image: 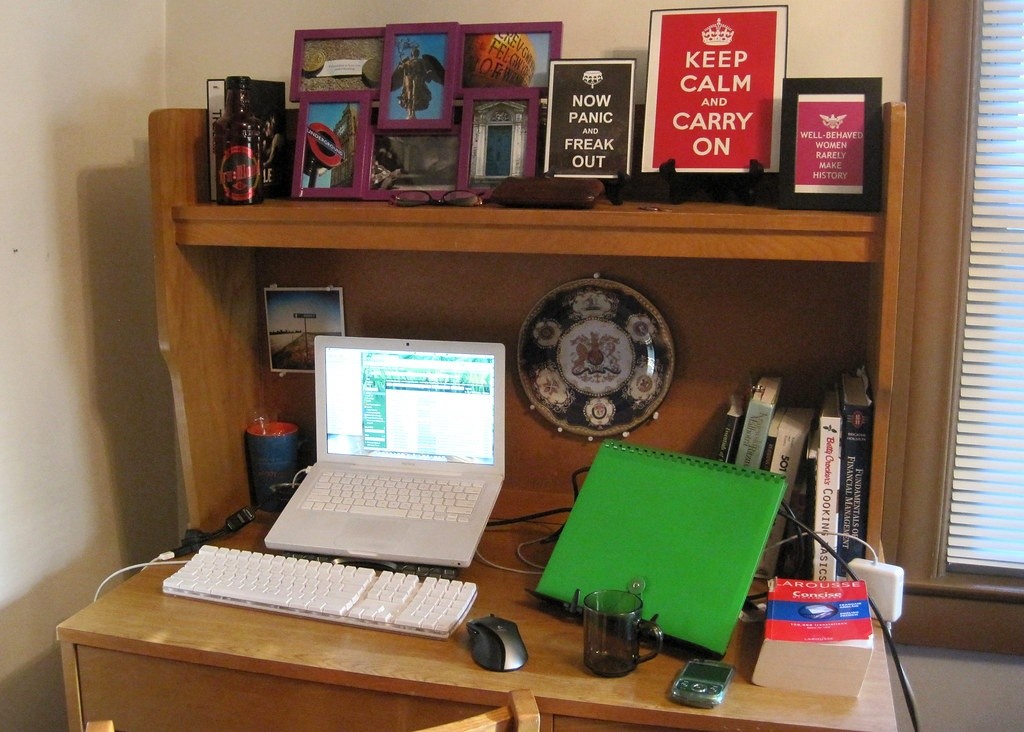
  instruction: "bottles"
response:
[214,75,264,204]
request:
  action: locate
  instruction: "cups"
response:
[247,421,298,513]
[584,590,663,678]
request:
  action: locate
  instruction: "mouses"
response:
[466,612,528,672]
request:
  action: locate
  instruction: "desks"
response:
[59,102,920,731]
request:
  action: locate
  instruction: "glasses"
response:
[388,189,484,207]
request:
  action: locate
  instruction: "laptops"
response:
[264,336,505,568]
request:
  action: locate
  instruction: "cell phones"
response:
[669,658,735,709]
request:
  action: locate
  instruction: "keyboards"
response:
[161,545,478,642]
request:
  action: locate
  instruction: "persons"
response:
[391,49,444,119]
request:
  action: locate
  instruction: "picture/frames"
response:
[291,6,881,213]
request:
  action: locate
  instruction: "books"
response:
[752,577,875,699]
[720,369,872,581]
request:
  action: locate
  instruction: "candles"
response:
[245,417,299,511]
